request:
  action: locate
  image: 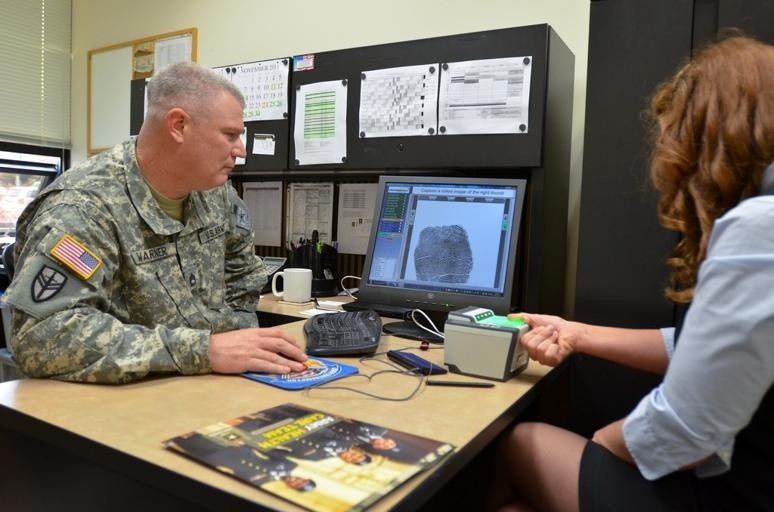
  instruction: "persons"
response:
[475,33,774,511]
[2,62,308,385]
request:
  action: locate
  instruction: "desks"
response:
[0,289,571,510]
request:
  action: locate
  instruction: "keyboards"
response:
[304,309,381,353]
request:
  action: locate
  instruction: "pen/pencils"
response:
[426,380,494,387]
[290,230,338,278]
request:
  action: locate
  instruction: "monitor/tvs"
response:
[344,176,533,339]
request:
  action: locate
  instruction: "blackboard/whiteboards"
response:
[87,28,197,153]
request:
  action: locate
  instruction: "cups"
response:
[271,268,313,305]
